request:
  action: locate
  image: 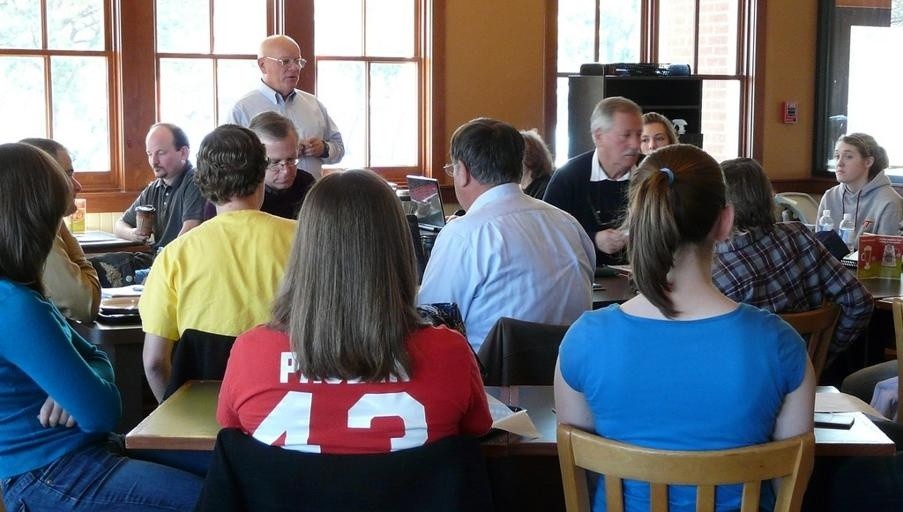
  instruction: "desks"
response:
[124,378,509,459]
[587,265,638,308]
[510,383,895,464]
[67,283,215,341]
[72,231,148,258]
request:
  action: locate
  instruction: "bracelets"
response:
[323,143,329,157]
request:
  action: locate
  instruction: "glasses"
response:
[444,162,457,177]
[268,56,306,69]
[590,205,626,228]
[266,157,298,171]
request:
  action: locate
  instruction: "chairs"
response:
[556,423,816,512]
[778,303,843,385]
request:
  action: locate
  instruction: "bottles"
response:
[399,196,411,216]
[819,209,834,231]
[839,214,854,253]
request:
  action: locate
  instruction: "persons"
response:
[0,143,215,512]
[232,34,345,182]
[814,132,902,250]
[414,117,598,357]
[444,129,557,224]
[638,111,679,157]
[137,123,299,405]
[87,123,203,288]
[16,138,103,327]
[552,144,817,511]
[217,166,492,454]
[709,157,875,372]
[541,96,650,266]
[203,112,317,220]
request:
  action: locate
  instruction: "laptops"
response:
[405,174,448,231]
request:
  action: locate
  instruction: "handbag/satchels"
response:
[416,302,489,379]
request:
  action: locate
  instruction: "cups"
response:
[861,250,872,270]
[134,205,155,237]
